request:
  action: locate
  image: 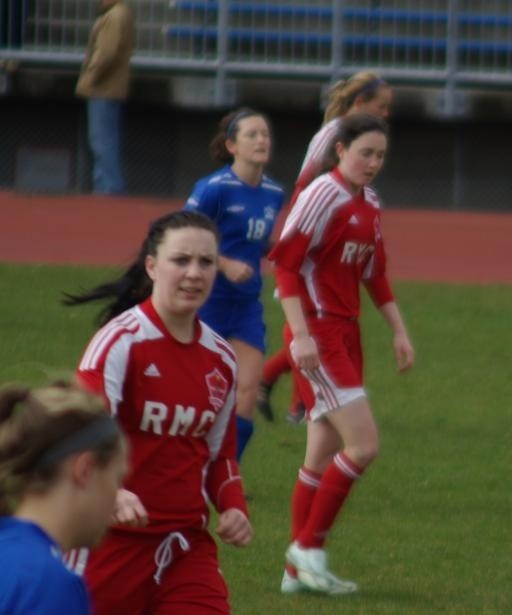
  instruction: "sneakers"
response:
[280,539,357,596]
[287,401,306,424]
[256,382,273,422]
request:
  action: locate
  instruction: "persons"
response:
[0,381,128,615]
[74,0,137,196]
[62,210,254,615]
[184,106,287,466]
